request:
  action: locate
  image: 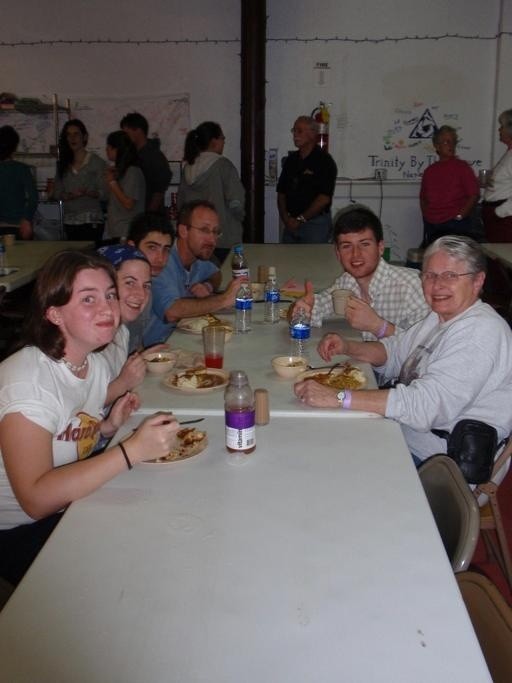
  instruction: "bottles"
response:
[478,168,492,184]
[0,236,9,272]
[231,248,311,365]
[224,368,270,454]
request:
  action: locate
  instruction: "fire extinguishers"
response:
[310,102,330,154]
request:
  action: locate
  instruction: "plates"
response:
[297,368,368,392]
[179,316,227,333]
[163,368,229,393]
[120,427,209,465]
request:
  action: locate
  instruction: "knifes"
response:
[131,417,207,431]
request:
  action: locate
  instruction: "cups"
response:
[203,325,224,368]
[6,234,15,246]
[332,288,355,314]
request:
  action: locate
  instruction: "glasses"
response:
[183,224,225,240]
[437,139,458,147]
[419,267,480,284]
[291,127,313,135]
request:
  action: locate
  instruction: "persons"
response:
[0,201,251,592]
[46,118,112,245]
[290,232,512,489]
[480,109,512,243]
[0,124,40,241]
[100,131,146,248]
[283,202,431,386]
[419,124,478,248]
[175,119,249,263]
[276,113,337,243]
[120,111,172,216]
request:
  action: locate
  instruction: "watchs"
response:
[336,387,345,408]
[455,214,464,221]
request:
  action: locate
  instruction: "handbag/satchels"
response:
[449,420,498,485]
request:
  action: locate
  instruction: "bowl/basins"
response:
[141,351,177,374]
[221,324,233,343]
[270,354,307,377]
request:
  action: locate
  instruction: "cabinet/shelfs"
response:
[0,91,72,238]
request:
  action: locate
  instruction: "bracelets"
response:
[296,214,306,224]
[375,319,388,338]
[343,387,352,409]
[109,180,117,185]
